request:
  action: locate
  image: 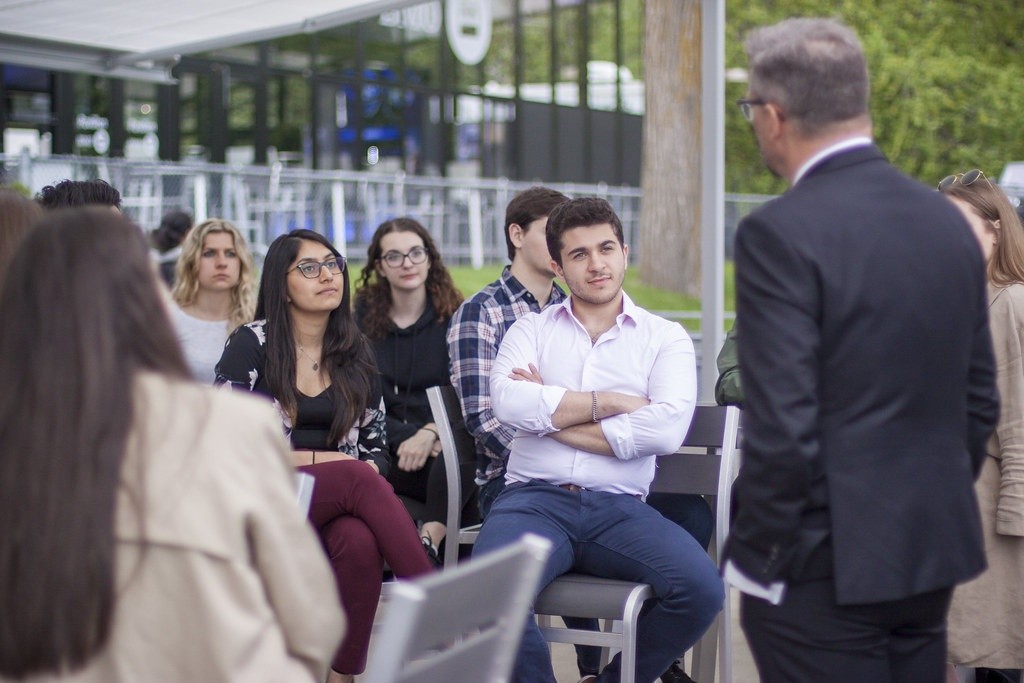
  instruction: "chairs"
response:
[358,384,738,683]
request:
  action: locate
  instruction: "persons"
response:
[0,177,724,683]
[712,167,1024,683]
[718,16,1001,683]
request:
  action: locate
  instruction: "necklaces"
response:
[297,345,321,371]
[592,324,615,344]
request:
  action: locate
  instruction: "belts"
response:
[559,484,590,493]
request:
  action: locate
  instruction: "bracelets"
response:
[313,450,315,464]
[591,389,600,423]
[419,427,438,439]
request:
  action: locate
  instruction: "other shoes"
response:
[660,661,699,683]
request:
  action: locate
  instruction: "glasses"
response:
[936,169,996,192]
[286,257,347,279]
[379,246,431,269]
[736,97,785,120]
